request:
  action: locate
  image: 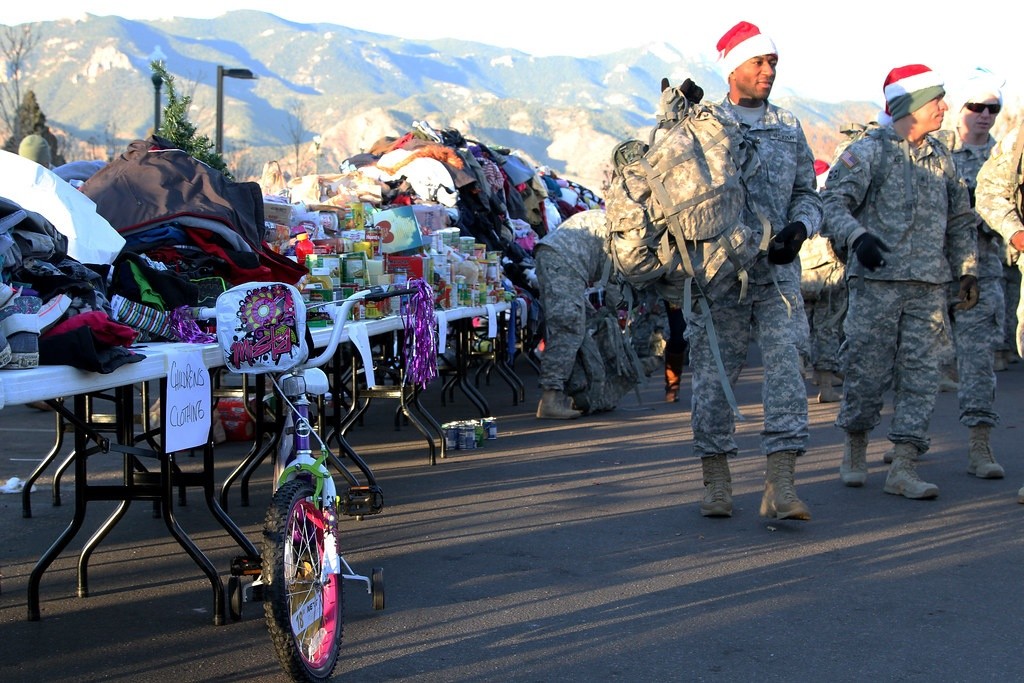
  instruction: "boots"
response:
[700,453,733,517]
[761,449,811,520]
[967,423,1005,477]
[798,349,1020,402]
[883,441,939,498]
[840,432,869,486]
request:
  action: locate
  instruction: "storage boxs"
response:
[218,397,257,420]
[264,202,292,224]
[305,251,389,327]
[221,420,255,441]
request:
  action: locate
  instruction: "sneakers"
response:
[0,283,72,371]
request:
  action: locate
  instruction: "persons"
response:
[660,22,824,519]
[975,127,1024,502]
[533,210,689,419]
[19,135,55,171]
[799,63,1008,496]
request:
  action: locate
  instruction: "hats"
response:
[814,159,832,178]
[877,64,944,126]
[956,68,1006,113]
[716,21,778,84]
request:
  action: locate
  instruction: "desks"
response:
[0,285,606,628]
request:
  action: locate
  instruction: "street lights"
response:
[214,66,254,156]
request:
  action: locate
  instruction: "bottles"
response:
[366,228,380,255]
[344,205,354,229]
[395,267,408,288]
[294,234,316,266]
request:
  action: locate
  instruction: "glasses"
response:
[964,102,1002,114]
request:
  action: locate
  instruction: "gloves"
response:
[955,276,980,311]
[768,221,807,264]
[853,232,891,273]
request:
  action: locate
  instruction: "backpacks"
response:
[600,139,676,290]
[568,313,640,413]
[831,121,882,173]
[643,110,740,240]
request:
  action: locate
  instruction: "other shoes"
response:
[884,443,896,462]
[536,393,579,419]
[665,389,677,403]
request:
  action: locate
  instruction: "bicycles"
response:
[169,278,425,682]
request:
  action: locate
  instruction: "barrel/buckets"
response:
[423,227,500,305]
[442,420,497,450]
[305,251,391,326]
